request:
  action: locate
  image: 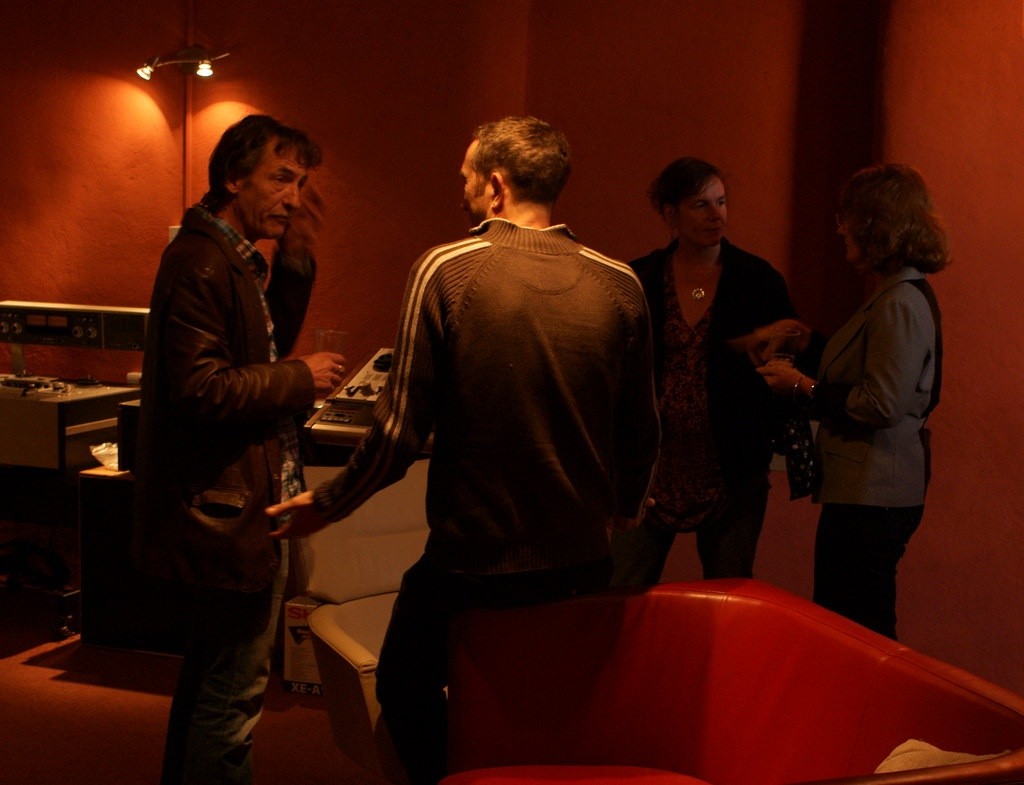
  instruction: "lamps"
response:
[136,43,213,81]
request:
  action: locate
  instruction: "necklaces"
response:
[691,287,704,302]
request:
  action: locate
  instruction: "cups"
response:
[769,328,800,369]
[315,330,350,374]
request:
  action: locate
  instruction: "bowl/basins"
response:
[88,446,119,472]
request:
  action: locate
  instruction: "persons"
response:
[747,163,954,639]
[141,115,346,785]
[265,112,662,785]
[618,158,815,593]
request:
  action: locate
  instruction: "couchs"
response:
[287,458,430,779]
[438,579,1024,785]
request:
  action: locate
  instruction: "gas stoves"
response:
[0,299,151,470]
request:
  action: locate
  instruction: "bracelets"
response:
[792,373,818,398]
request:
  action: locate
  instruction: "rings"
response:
[337,364,345,376]
